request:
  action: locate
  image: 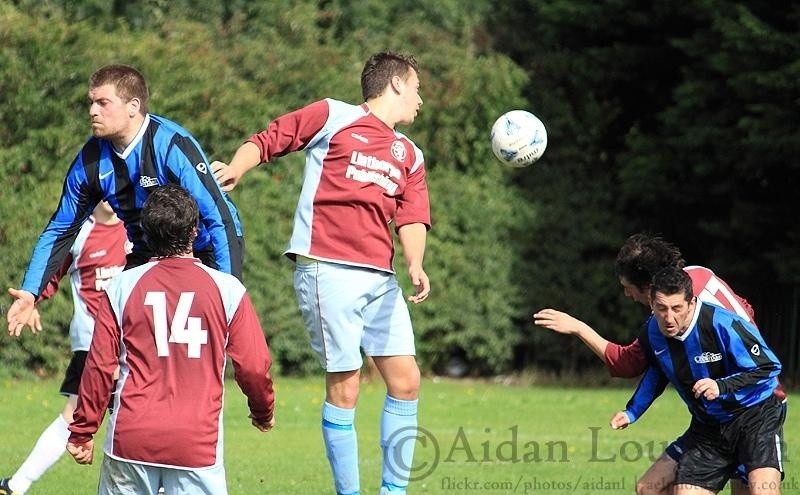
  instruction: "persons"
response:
[209,52,434,495]
[609,261,787,495]
[532,232,790,495]
[1,194,136,495]
[66,188,276,495]
[3,66,244,341]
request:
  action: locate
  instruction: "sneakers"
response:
[0,477,20,495]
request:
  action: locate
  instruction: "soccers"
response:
[491,110,547,169]
